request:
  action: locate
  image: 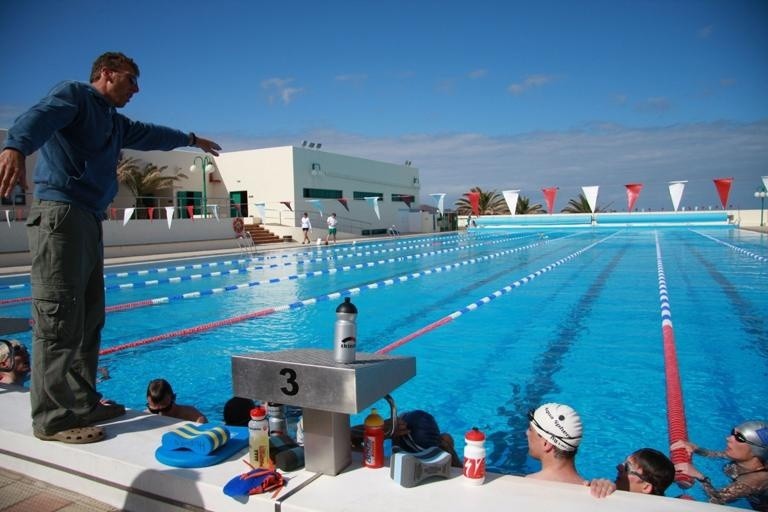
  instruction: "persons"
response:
[0,339,30,388]
[299,211,312,244]
[521,403,584,485]
[222,397,256,426]
[324,213,337,243]
[96,378,209,425]
[668,420,767,512]
[348,409,461,468]
[0,51,221,446]
[582,448,675,500]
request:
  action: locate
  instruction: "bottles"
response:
[364,407,385,467]
[285,405,303,440]
[335,295,357,363]
[249,408,271,465]
[267,400,287,437]
[275,444,305,471]
[462,426,486,485]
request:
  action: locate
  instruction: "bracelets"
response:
[190,130,195,148]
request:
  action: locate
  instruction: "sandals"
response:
[33,424,108,443]
[83,403,126,423]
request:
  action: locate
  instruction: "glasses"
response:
[624,461,645,481]
[146,392,175,415]
[731,427,763,449]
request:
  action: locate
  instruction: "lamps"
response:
[414,179,420,185]
[308,164,326,177]
[298,140,323,150]
[402,160,409,165]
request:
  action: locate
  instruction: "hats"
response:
[737,420,768,457]
[530,402,581,451]
[398,411,440,455]
